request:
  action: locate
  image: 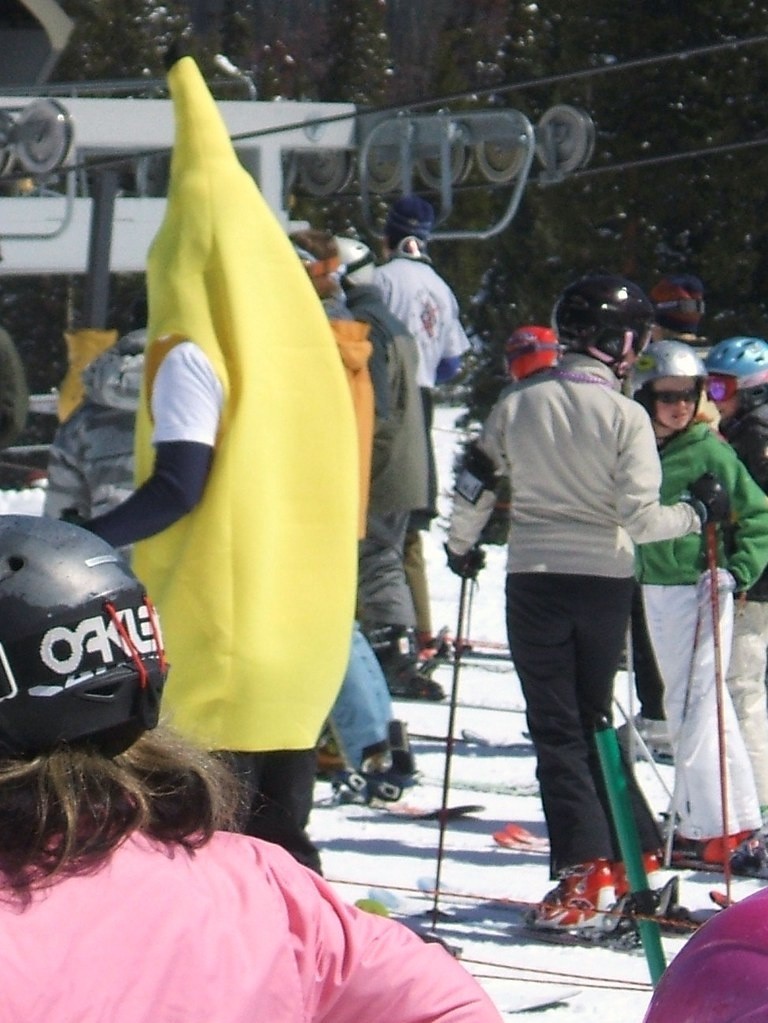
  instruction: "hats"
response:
[385,197,435,264]
[648,273,704,331]
[289,230,340,298]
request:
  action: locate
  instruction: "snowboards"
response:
[375,800,488,822]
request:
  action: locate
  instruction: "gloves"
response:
[686,472,730,521]
[444,541,487,578]
[694,567,735,605]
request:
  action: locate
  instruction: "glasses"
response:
[707,377,739,402]
[654,389,701,404]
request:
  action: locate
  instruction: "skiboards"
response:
[365,875,696,960]
[461,728,676,767]
[490,821,768,880]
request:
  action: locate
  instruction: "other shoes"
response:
[317,736,382,776]
[619,715,671,754]
[370,626,446,700]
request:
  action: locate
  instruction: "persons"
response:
[43,293,152,565]
[1,512,505,1022]
[445,272,732,931]
[63,55,362,881]
[282,196,562,800]
[619,275,767,867]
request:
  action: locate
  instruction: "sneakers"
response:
[663,830,754,869]
[521,854,668,932]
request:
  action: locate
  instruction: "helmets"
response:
[623,340,708,416]
[706,336,768,387]
[504,327,556,379]
[553,275,651,362]
[0,514,171,758]
[337,238,376,287]
